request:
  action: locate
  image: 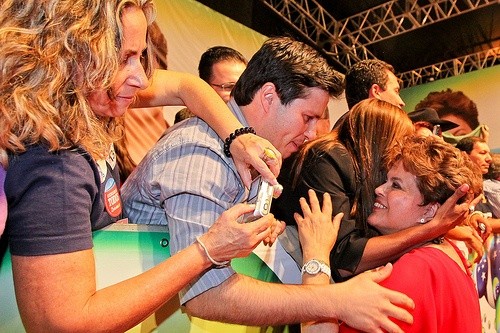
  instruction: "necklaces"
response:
[430,236,445,245]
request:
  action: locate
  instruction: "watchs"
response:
[300,259,331,280]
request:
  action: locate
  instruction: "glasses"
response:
[211,83,236,93]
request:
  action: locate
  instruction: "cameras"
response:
[243,174,283,223]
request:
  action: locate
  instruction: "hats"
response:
[407,108,460,132]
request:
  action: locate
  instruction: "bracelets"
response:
[223,126,257,158]
[195,232,232,267]
[467,210,483,228]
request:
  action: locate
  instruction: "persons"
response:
[415,87,500,180]
[121,0,500,333]
[0,0,282,333]
